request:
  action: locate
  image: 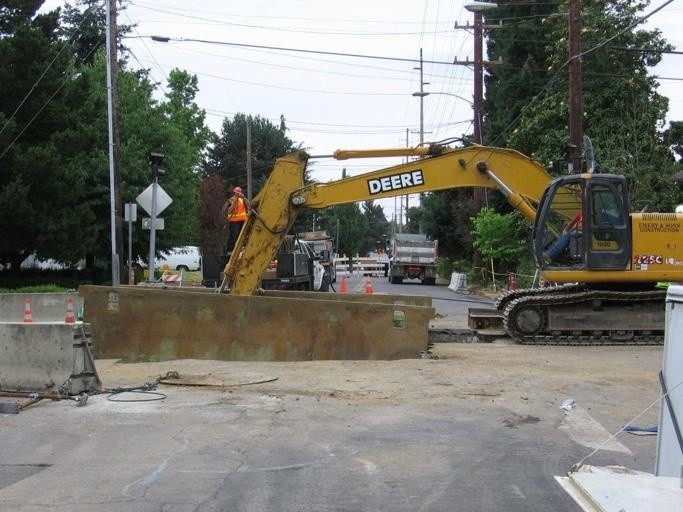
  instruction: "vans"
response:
[133,246,200,272]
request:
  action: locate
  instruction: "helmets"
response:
[233,187,242,195]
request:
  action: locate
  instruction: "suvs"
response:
[364,253,388,277]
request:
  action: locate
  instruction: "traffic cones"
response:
[339,277,348,293]
[22,295,34,323]
[363,274,373,293]
[510,273,516,291]
[63,296,76,323]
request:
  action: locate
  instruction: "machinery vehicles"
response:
[213,135,683,345]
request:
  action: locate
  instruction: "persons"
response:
[226,186,247,250]
[543,209,583,259]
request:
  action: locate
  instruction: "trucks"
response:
[387,232,439,285]
[196,236,332,295]
[296,232,336,284]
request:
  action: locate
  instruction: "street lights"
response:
[146,150,165,283]
[462,0,585,173]
[411,91,484,273]
